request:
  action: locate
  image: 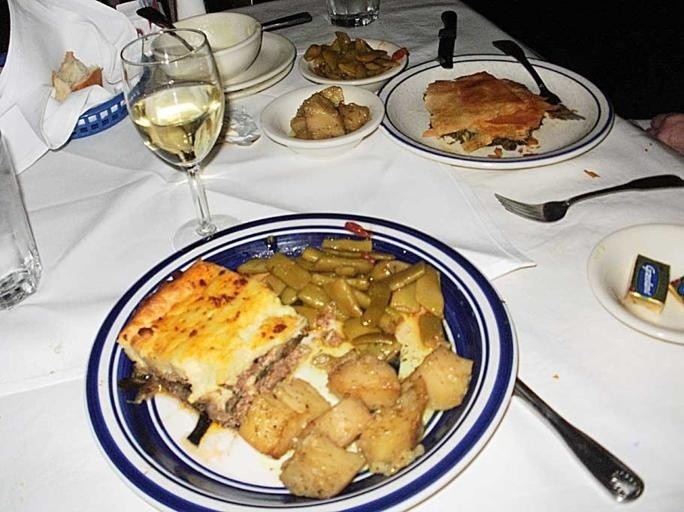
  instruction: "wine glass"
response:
[117,27,240,253]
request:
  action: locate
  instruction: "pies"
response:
[112,259,310,434]
[419,72,553,156]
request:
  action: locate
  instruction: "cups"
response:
[0,134,41,309]
[325,2,379,28]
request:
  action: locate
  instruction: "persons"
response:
[647,113,683,156]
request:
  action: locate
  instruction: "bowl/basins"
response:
[152,12,266,86]
[300,35,409,96]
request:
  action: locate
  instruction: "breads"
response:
[52,50,102,103]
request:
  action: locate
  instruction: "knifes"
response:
[435,9,458,72]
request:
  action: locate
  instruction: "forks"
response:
[492,172,683,224]
[490,36,584,126]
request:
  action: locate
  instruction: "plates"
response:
[84,212,522,512]
[208,63,298,102]
[213,32,296,91]
[378,54,616,177]
[258,83,390,158]
[581,226,684,346]
